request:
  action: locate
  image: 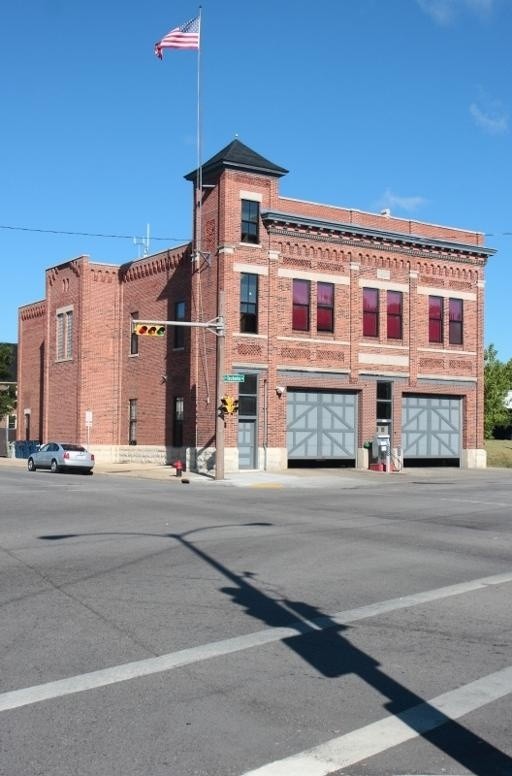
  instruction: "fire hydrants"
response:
[172,459,183,476]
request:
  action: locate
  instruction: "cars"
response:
[26,440,95,472]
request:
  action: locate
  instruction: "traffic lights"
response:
[231,397,239,412]
[135,323,165,335]
[220,395,232,413]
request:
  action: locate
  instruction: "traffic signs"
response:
[223,373,244,381]
